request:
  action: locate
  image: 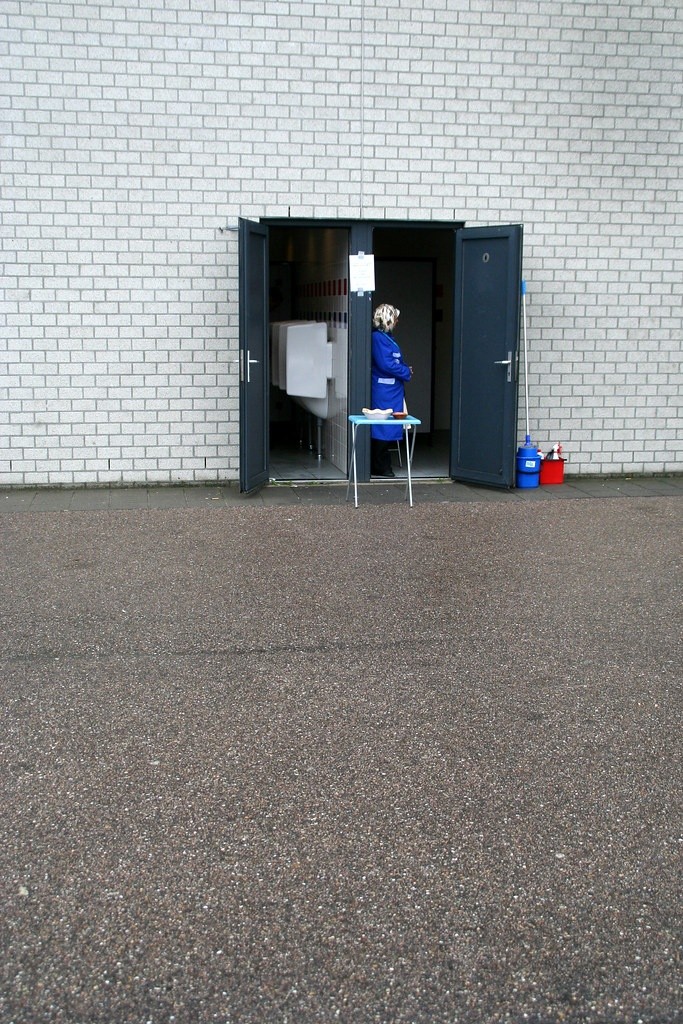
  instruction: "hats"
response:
[373,304,400,331]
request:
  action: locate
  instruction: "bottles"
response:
[537,449,544,459]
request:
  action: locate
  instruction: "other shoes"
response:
[385,472,394,477]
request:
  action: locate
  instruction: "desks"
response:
[346,416,421,508]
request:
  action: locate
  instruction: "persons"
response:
[372,303,414,477]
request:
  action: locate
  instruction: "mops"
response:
[520,280,538,458]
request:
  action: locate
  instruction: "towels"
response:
[370,417,403,441]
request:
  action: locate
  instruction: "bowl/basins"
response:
[393,412,407,419]
[361,408,393,420]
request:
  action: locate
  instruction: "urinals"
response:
[301,383,337,418]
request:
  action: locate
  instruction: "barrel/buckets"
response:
[516,456,542,488]
[540,458,564,484]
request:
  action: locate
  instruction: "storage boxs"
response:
[539,458,568,485]
